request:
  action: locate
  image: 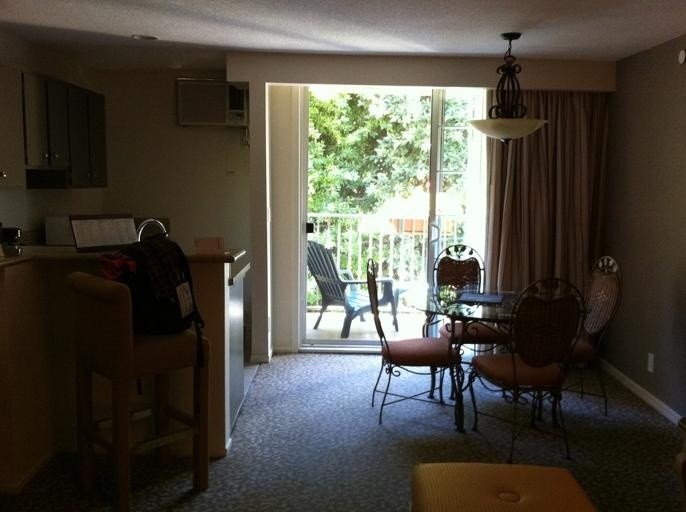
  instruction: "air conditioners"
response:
[178,79,248,131]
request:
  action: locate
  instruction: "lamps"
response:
[470,32,551,144]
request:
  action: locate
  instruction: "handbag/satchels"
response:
[115,219,197,335]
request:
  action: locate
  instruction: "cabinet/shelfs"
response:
[22,71,69,169]
[68,85,106,186]
[0,65,26,190]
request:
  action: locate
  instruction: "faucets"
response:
[136,217,168,241]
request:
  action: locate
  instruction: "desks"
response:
[408,296,592,432]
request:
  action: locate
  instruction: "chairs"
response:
[68,273,211,511]
[431,244,487,402]
[366,257,462,428]
[467,276,587,464]
[306,242,401,339]
[537,254,623,429]
